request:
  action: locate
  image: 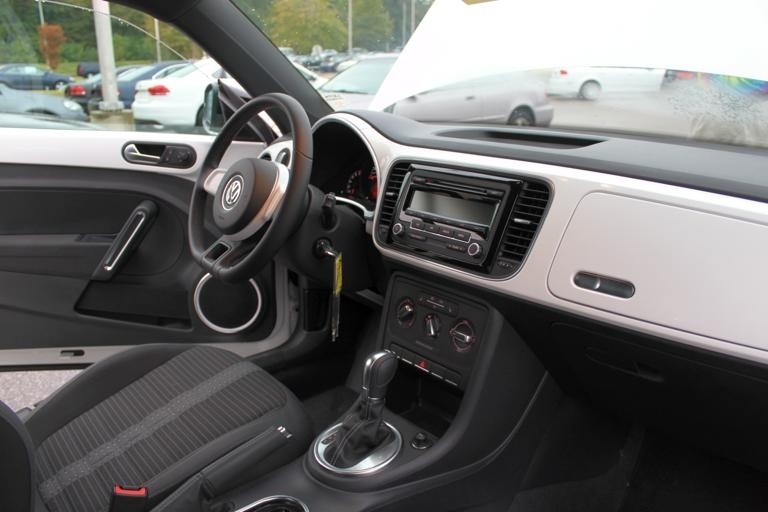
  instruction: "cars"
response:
[315,55,552,126]
[66,59,328,128]
[1,60,73,89]
[283,46,371,72]
[547,66,666,102]
[0,84,87,123]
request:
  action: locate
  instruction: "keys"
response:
[316,240,340,259]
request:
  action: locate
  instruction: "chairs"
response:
[0,344,316,512]
